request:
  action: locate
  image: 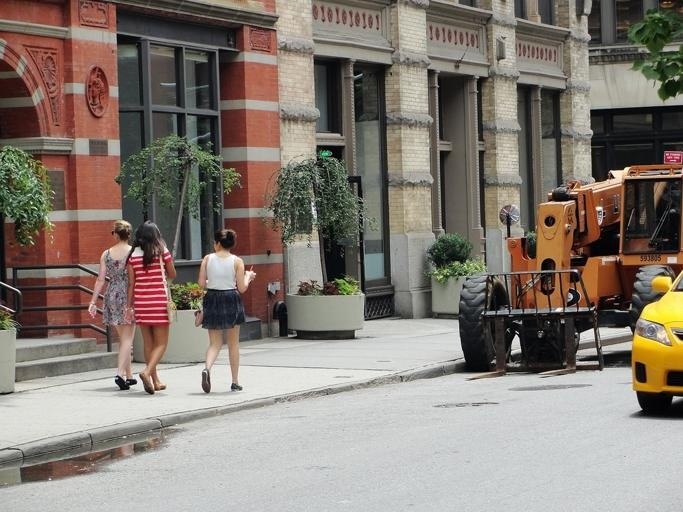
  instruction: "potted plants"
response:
[0,145,55,393]
[424,233,486,318]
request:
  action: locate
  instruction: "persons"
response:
[87,218,140,391]
[120,219,176,396]
[196,227,257,393]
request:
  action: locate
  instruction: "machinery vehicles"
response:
[459,164,683,380]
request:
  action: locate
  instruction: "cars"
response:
[631,270,683,411]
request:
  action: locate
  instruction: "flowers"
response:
[295,276,362,296]
[170,282,204,309]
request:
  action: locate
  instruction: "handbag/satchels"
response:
[192,307,204,328]
[166,298,177,323]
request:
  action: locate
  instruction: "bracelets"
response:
[89,302,97,306]
[124,306,133,312]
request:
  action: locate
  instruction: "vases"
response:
[286,294,365,340]
[133,310,218,362]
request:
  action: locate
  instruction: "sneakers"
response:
[125,377,137,384]
[229,383,242,392]
[199,367,211,393]
[137,371,167,394]
[113,375,130,391]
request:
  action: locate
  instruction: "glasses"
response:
[110,228,115,236]
